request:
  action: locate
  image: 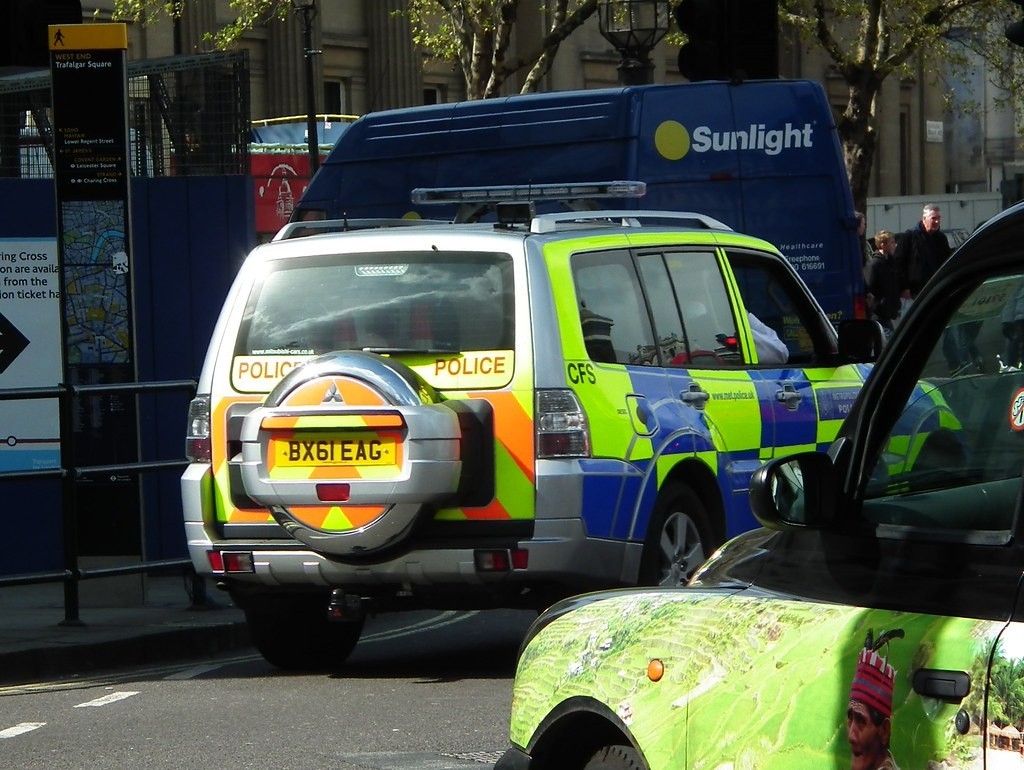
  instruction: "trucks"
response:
[273,76,871,360]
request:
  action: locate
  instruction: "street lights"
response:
[598,0,675,87]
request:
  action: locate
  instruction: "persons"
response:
[745,311,790,365]
[867,230,904,340]
[895,201,951,303]
[847,650,897,770]
[855,210,885,322]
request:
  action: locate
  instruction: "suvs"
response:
[174,178,961,655]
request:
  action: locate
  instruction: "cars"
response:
[492,198,1022,768]
